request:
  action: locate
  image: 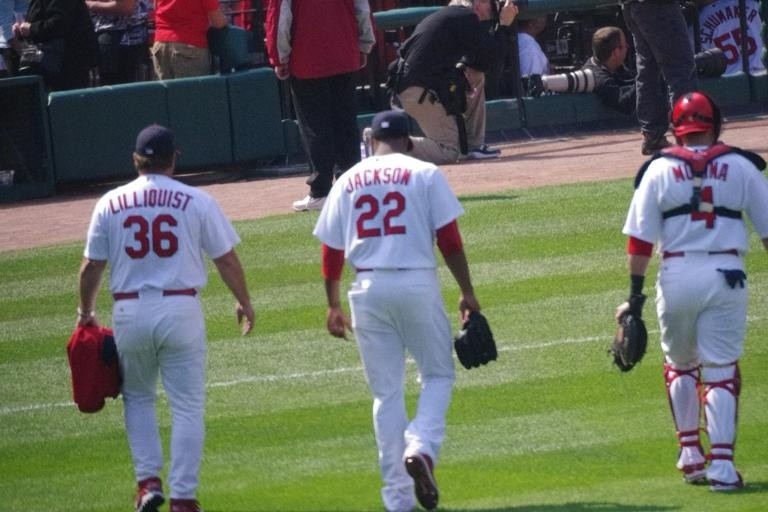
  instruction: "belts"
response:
[113,288,197,299]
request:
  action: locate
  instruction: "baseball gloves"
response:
[609,313,647,372]
[454,309,497,368]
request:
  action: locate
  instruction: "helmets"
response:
[669,92,717,137]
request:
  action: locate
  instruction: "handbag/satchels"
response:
[19,42,57,82]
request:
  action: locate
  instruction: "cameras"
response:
[489,0,530,12]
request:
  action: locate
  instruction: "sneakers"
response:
[405,454,439,510]
[683,463,706,482]
[362,128,373,157]
[710,478,743,491]
[642,136,673,154]
[135,477,164,512]
[170,498,201,512]
[458,144,500,160]
[292,195,326,211]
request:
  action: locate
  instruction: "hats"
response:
[371,111,409,136]
[135,124,181,159]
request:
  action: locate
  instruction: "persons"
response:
[267,1,374,212]
[615,92,767,492]
[365,1,696,166]
[75,124,256,512]
[312,111,481,511]
[1,0,267,90]
